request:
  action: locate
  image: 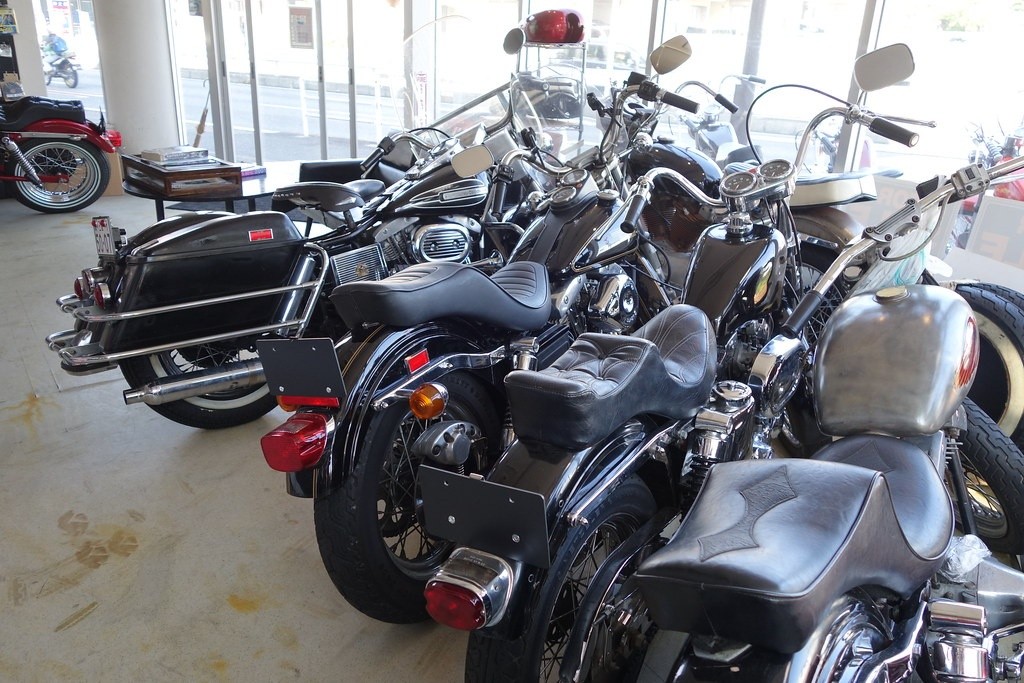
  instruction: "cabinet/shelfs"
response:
[0,35,20,82]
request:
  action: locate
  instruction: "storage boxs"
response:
[45,152,124,196]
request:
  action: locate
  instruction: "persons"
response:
[42,26,66,75]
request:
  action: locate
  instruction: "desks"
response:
[121,159,308,223]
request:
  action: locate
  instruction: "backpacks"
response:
[50,37,67,55]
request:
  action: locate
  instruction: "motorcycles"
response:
[0,96,121,215]
[43,28,1024,683]
[40,44,78,89]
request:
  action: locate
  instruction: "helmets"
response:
[517,75,582,118]
[521,8,585,44]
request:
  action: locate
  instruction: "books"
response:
[232,163,266,176]
[131,145,232,189]
